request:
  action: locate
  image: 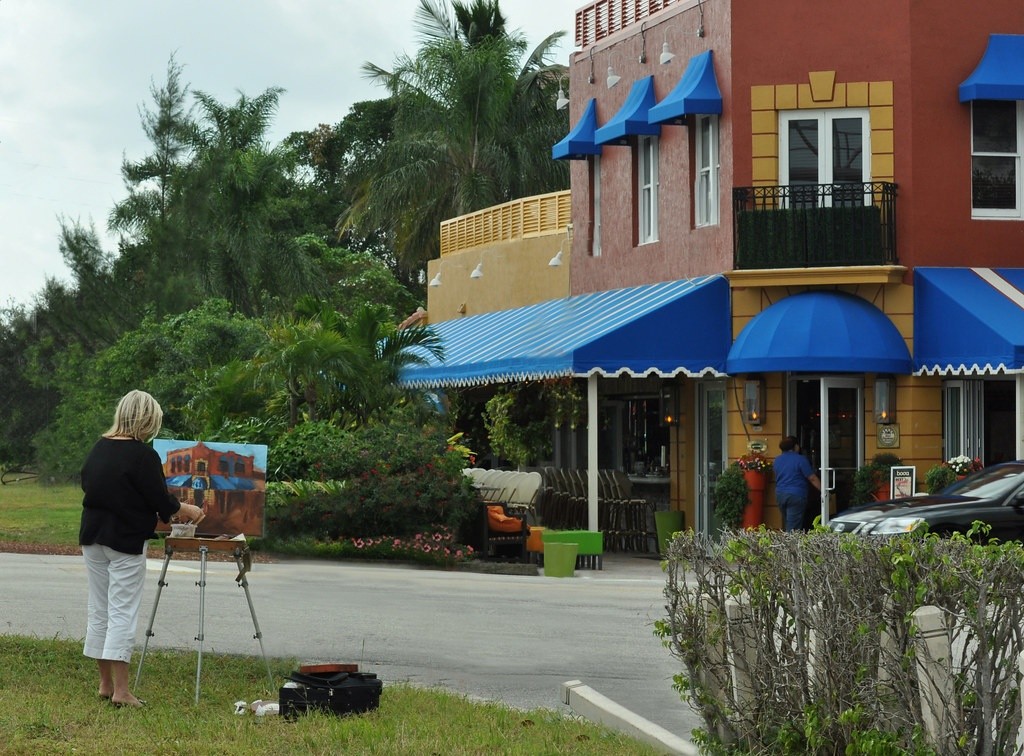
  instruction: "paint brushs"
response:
[174,501,206,537]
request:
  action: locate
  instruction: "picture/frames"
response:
[890,465,916,500]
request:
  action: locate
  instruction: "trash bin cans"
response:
[653,510,684,557]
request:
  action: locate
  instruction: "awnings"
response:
[911,266,1024,375]
[551,98,603,161]
[594,75,660,146]
[319,273,733,389]
[728,291,912,381]
[958,34,1024,103]
[647,49,723,126]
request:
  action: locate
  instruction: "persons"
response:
[774,436,821,536]
[80,389,204,706]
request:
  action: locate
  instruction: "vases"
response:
[956,476,966,481]
[742,470,766,531]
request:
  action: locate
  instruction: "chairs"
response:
[459,467,660,571]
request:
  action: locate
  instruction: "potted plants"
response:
[872,452,903,502]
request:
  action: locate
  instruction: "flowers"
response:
[942,455,983,475]
[738,447,772,474]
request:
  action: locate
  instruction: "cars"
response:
[822,456,1024,552]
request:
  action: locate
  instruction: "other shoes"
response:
[98,691,114,700]
[116,699,148,709]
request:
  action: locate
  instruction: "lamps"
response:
[430,261,446,288]
[659,25,703,65]
[470,251,488,279]
[548,238,568,266]
[742,380,766,426]
[873,378,896,423]
[659,383,680,427]
[607,50,646,89]
[557,74,594,110]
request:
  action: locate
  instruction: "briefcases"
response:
[279,670,383,724]
